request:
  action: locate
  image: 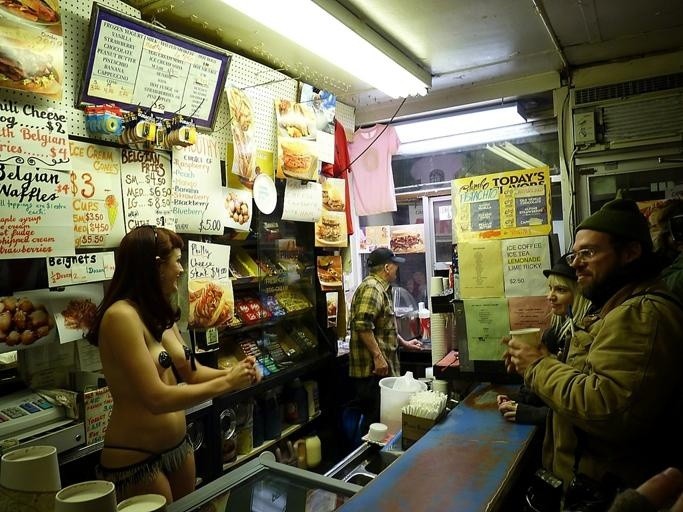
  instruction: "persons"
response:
[495,252,590,425]
[500,198,681,512]
[604,467,682,512]
[82,224,260,511]
[349,247,425,440]
[651,202,683,303]
[404,264,425,301]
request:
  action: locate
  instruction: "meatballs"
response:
[225,191,249,224]
[0,295,54,345]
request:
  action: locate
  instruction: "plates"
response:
[253,173,278,215]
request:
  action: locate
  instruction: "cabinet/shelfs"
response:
[176,221,337,483]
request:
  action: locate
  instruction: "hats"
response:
[366,247,405,266]
[572,200,652,248]
[542,253,578,280]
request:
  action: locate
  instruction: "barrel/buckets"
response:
[378,375,430,436]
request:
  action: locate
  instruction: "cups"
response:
[429,276,453,396]
[366,421,389,443]
[509,327,541,351]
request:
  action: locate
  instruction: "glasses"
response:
[132,224,158,245]
[565,246,613,268]
